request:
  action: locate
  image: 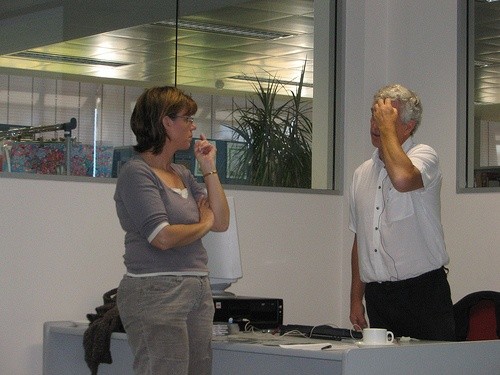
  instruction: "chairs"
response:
[450,291,500,341]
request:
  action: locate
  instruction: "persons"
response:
[114,87,230,375]
[347,83,454,342]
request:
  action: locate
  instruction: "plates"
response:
[355,342,395,348]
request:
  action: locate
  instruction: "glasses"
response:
[166,114,195,126]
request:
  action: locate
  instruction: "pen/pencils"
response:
[321,345,331,350]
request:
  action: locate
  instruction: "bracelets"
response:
[204,171,217,177]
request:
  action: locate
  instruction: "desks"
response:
[42,320,500,375]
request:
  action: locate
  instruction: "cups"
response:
[362,328,394,344]
